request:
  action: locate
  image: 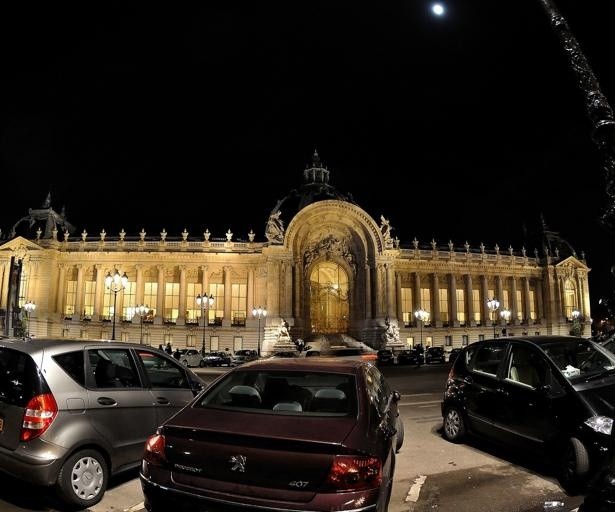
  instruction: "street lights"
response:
[571,309,581,337]
[103,267,131,341]
[487,296,500,339]
[195,292,215,358]
[134,303,153,345]
[251,305,268,357]
[500,308,512,337]
[414,306,431,354]
[23,300,37,339]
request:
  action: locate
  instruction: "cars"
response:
[139,351,410,512]
[167,344,466,367]
[441,334,615,496]
[1,339,207,509]
[578,334,615,373]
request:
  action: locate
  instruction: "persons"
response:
[159,343,180,360]
